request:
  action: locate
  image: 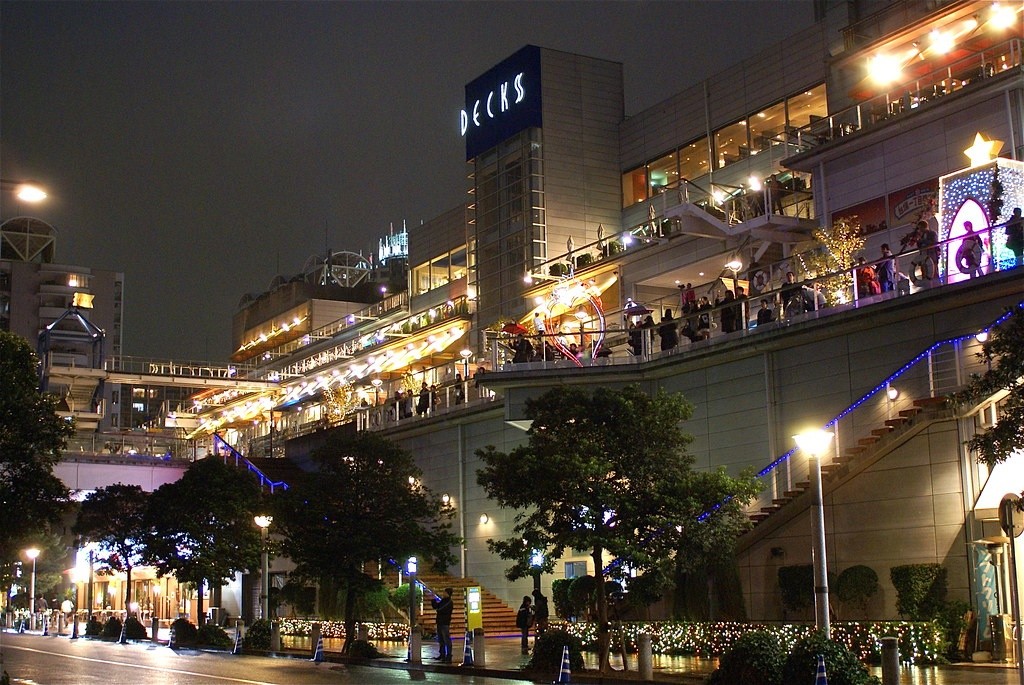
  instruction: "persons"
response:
[629,271,827,363]
[516,588,549,655]
[963,221,984,279]
[315,367,486,432]
[850,242,908,299]
[769,174,785,215]
[916,220,941,291]
[431,588,454,661]
[1005,208,1024,266]
[34,593,74,628]
[508,313,596,364]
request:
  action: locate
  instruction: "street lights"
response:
[724,259,743,298]
[790,426,836,649]
[460,349,473,380]
[574,303,589,346]
[253,510,274,624]
[25,548,40,631]
[370,379,384,407]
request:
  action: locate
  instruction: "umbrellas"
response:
[501,321,529,339]
[566,235,574,269]
[596,223,606,253]
[624,305,653,315]
[648,203,656,240]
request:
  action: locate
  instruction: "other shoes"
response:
[433,654,453,664]
